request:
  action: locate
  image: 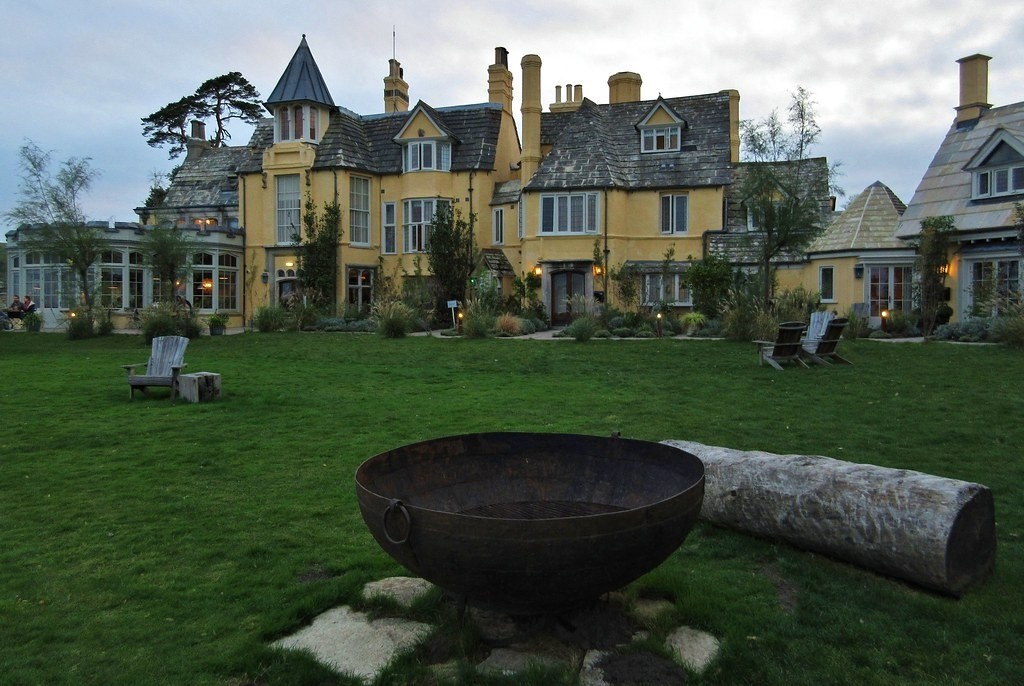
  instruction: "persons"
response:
[22,295,35,315]
[9,295,23,310]
[177,295,191,311]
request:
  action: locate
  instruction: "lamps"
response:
[262,273,268,283]
[853,264,864,278]
[535,264,542,276]
[593,261,603,274]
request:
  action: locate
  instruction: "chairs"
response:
[120,336,191,403]
[9,318,22,329]
[752,322,810,371]
[802,317,854,367]
[801,310,834,352]
[50,308,68,332]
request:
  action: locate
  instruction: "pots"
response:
[354,431,704,614]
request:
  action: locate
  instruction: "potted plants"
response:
[202,309,230,336]
[19,311,45,334]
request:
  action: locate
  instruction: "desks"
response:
[178,372,222,403]
